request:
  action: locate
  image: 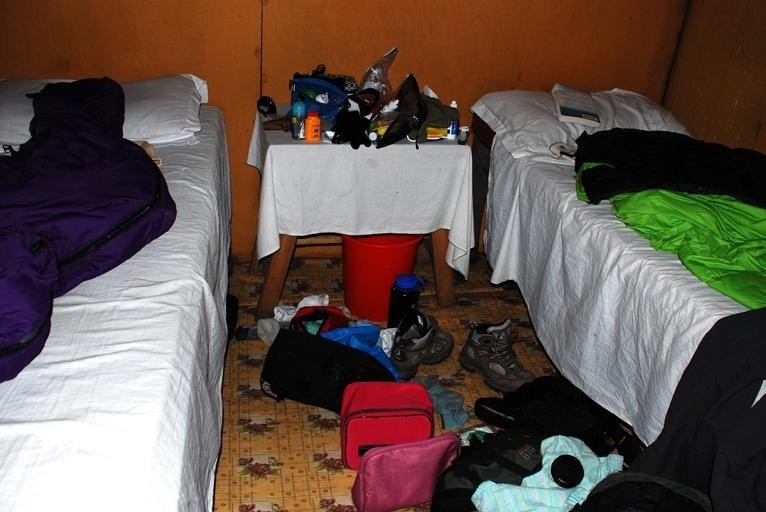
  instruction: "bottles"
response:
[389,273,425,330]
[291,101,322,144]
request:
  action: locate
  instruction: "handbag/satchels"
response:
[350,431,461,512]
[292,305,348,337]
[407,94,460,143]
[340,381,436,471]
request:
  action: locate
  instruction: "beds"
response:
[479,136,766,448]
[0,103,232,511]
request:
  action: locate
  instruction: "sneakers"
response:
[389,310,455,382]
[457,319,537,395]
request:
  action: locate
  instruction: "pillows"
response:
[471,88,696,160]
[0,73,210,150]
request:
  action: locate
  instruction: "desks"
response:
[253,102,473,321]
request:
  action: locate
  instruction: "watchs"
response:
[254,95,279,117]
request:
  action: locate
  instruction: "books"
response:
[551,82,601,128]
